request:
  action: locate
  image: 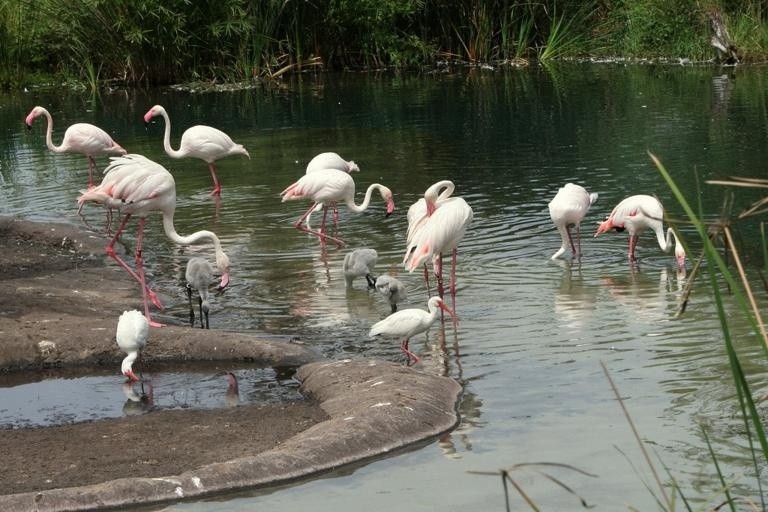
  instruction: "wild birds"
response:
[594,193,687,280]
[280,152,397,249]
[76,153,232,329]
[144,105,251,196]
[368,296,454,365]
[25,105,127,187]
[374,273,409,313]
[115,309,151,381]
[185,256,213,320]
[548,182,599,260]
[342,249,378,291]
[403,180,474,295]
